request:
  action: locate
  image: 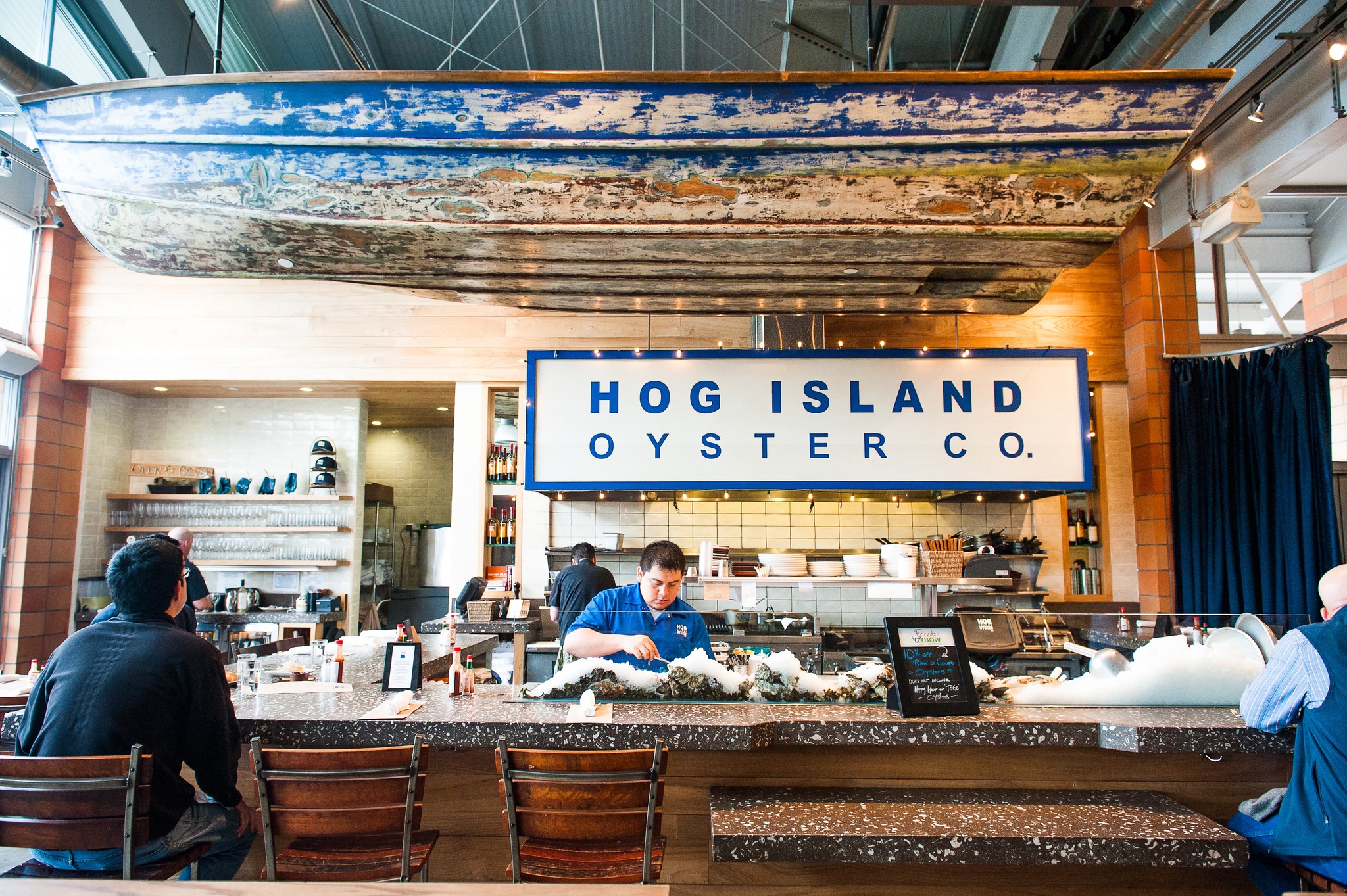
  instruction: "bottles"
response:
[1067,507,1099,546]
[748,649,768,676]
[1117,607,1209,646]
[237,579,248,614]
[28,659,45,684]
[320,615,475,698]
[487,442,515,545]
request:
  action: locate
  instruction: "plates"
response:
[225,667,316,686]
[260,607,292,611]
[731,545,880,577]
[712,544,730,576]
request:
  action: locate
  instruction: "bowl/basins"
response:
[711,643,727,647]
[764,619,854,650]
[880,544,919,578]
[225,587,260,613]
[728,647,770,658]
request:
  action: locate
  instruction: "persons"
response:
[1224,565,1347,887]
[10,538,257,881]
[167,526,214,635]
[547,542,616,682]
[563,541,716,674]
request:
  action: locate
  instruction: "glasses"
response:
[180,567,191,578]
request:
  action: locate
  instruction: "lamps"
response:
[1245,97,1267,123]
[1188,142,1208,171]
[1143,192,1158,208]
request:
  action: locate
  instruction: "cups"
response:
[236,639,329,699]
[290,671,309,681]
[897,558,916,578]
[108,510,132,558]
[270,512,350,560]
[729,664,749,675]
[686,541,729,576]
[296,591,322,612]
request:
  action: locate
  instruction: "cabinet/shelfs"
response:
[307,435,339,495]
[100,493,353,570]
[484,382,523,600]
[359,501,397,605]
[1060,383,1114,603]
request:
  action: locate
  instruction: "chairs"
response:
[263,630,305,652]
[491,732,671,887]
[1279,852,1347,896]
[0,743,216,882]
[246,732,444,884]
[231,636,278,664]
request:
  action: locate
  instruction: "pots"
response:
[723,596,768,627]
[876,528,1043,555]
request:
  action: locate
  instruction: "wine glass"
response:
[132,502,272,560]
[210,592,221,613]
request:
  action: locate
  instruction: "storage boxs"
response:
[364,481,394,504]
[919,550,966,579]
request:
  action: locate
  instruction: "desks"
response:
[74,622,346,666]
